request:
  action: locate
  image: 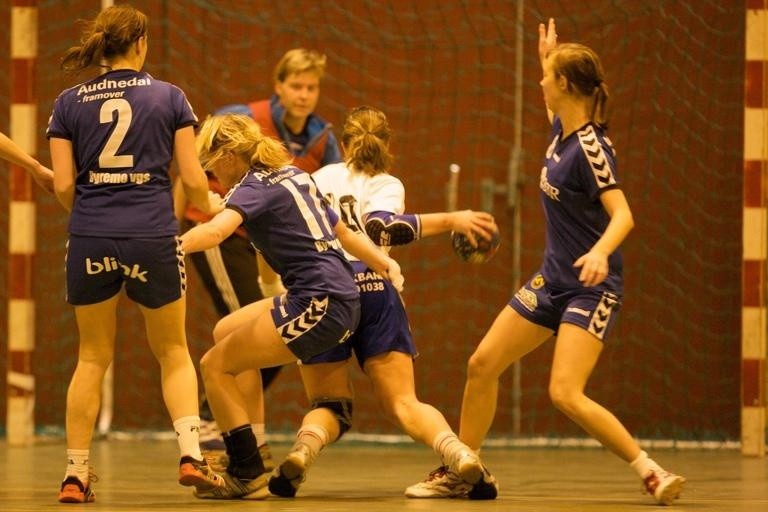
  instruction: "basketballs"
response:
[450,219,502,268]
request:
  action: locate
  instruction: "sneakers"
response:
[58,467,99,502]
[639,467,686,505]
[179,413,315,501]
[404,443,499,499]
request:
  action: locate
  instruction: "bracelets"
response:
[29,160,39,174]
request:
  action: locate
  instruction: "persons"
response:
[171,48,342,452]
[268,104,501,502]
[0,130,56,192]
[403,16,687,506]
[42,4,225,503]
[179,111,365,501]
